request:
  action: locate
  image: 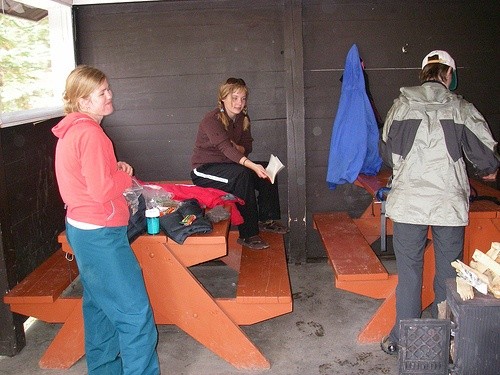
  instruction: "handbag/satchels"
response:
[127,193,145,243]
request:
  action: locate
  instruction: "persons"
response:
[380,49,500,361]
[52,66,163,375]
[189,77,288,249]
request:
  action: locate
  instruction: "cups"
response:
[145,208,160,235]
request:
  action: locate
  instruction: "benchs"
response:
[215,226,294,327]
[312,208,399,300]
[3,248,80,323]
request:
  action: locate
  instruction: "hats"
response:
[422,50,457,91]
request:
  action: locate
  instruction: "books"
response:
[260,155,285,184]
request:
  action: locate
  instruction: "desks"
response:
[36,181,272,369]
[353,169,500,342]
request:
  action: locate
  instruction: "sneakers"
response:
[258,219,290,234]
[381,336,397,355]
[238,235,270,249]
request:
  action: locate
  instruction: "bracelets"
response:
[243,158,249,166]
